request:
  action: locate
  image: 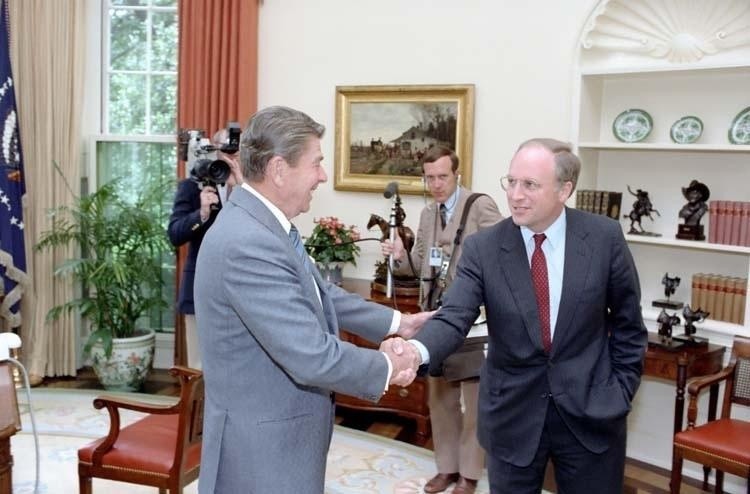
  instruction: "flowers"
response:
[303,215,360,268]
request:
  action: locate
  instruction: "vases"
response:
[314,260,344,285]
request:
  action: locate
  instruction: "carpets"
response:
[10,387,553,494]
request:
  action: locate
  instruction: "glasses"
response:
[499,176,542,193]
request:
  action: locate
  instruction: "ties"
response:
[288,223,313,277]
[440,204,447,230]
[530,233,551,352]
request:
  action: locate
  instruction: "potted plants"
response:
[35,154,174,391]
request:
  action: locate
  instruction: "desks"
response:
[317,275,441,445]
[608,332,726,494]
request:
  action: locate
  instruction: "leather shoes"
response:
[425,471,459,493]
[451,474,478,494]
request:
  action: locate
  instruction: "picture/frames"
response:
[334,84,473,195]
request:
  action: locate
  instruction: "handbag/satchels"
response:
[443,306,489,383]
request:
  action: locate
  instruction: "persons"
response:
[167,127,248,371]
[192,105,439,494]
[381,137,649,494]
[380,145,509,494]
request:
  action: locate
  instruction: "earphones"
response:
[456,174,461,186]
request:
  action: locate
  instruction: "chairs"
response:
[78,366,203,494]
[674,334,749,494]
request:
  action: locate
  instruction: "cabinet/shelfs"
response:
[570,67,749,347]
